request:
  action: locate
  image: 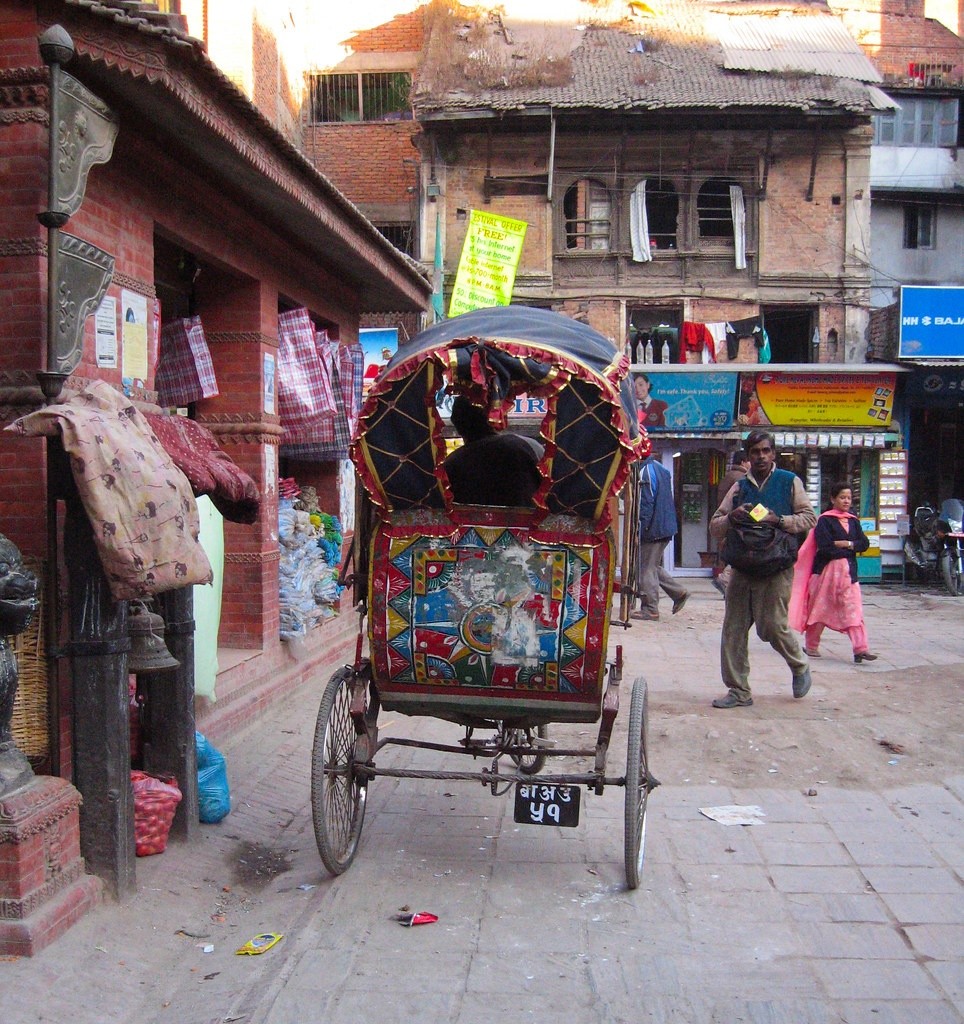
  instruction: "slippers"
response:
[631,611,659,621]
[671,589,693,613]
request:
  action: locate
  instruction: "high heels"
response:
[855,650,877,663]
[805,647,821,657]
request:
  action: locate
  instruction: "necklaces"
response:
[840,518,848,523]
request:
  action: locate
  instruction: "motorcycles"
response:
[904,499,964,597]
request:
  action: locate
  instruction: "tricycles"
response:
[308,304,663,890]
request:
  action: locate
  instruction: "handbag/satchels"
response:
[195,731,231,823]
[279,307,365,462]
[717,507,799,575]
[156,315,219,408]
[127,768,180,857]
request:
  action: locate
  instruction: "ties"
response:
[640,402,647,413]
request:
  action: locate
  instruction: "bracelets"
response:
[848,541,852,549]
[781,518,784,524]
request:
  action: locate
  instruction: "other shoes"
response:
[711,578,725,593]
[712,693,753,708]
[793,661,813,698]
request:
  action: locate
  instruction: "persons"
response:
[709,429,817,708]
[634,374,668,427]
[788,481,877,662]
[442,394,546,507]
[630,454,690,621]
